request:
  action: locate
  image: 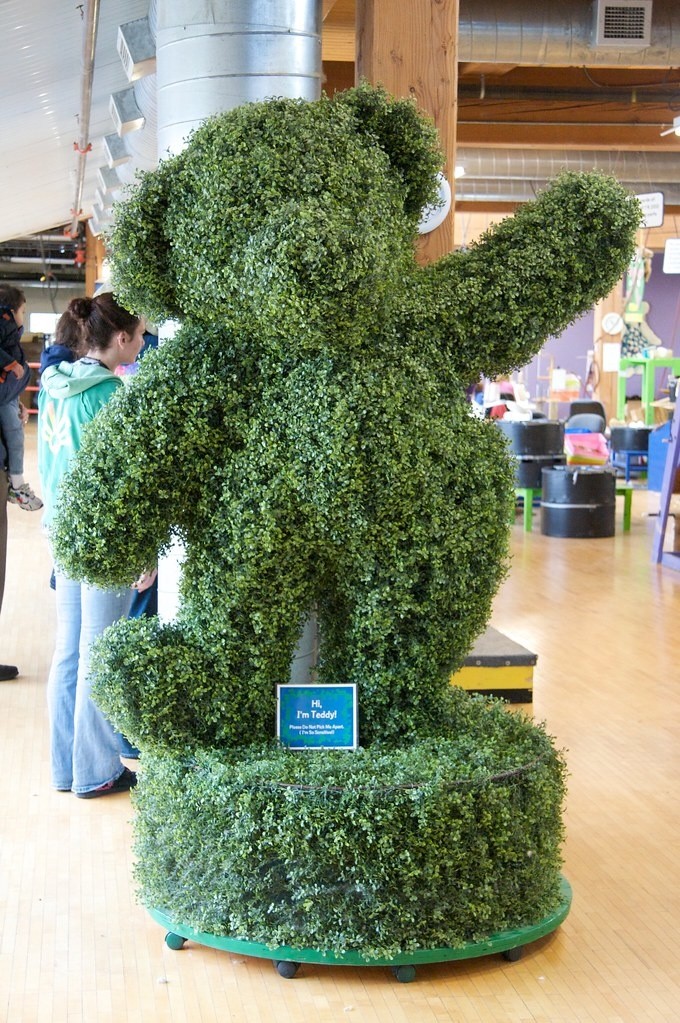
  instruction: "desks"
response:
[612,449,648,483]
[510,488,634,532]
[617,357,679,425]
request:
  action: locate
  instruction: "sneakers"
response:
[7,483,44,511]
[76,766,138,797]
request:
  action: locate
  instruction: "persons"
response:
[0,284,44,511]
[36,292,156,798]
[0,401,30,682]
[112,318,157,782]
[40,311,83,590]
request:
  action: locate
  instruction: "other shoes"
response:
[0,665,19,681]
